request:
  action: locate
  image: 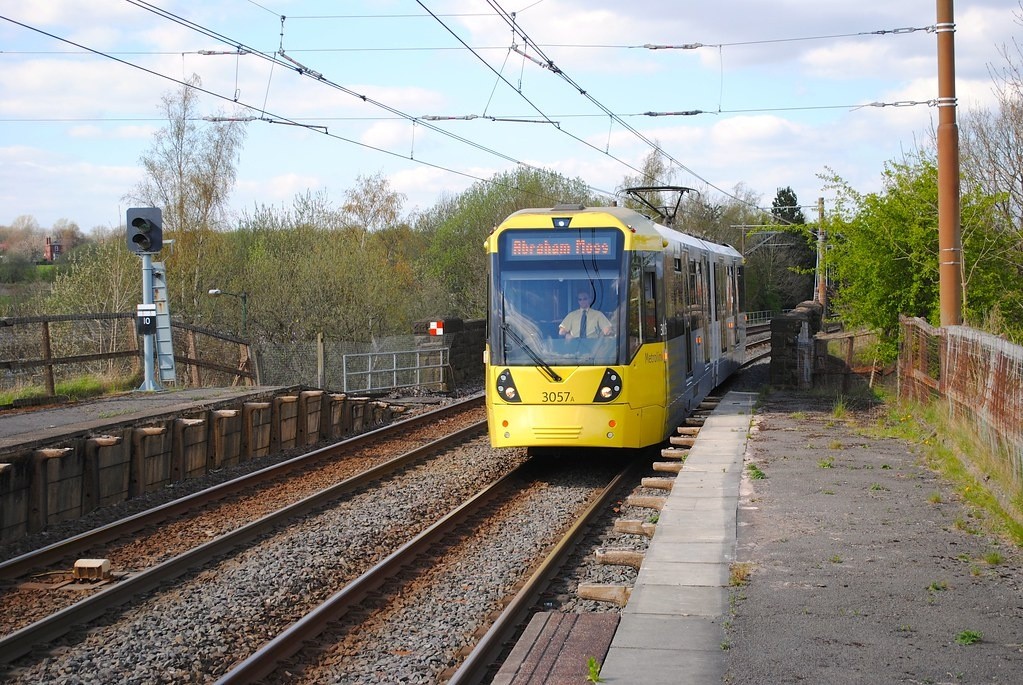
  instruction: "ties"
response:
[580,310,587,338]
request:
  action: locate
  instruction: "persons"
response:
[559,291,612,339]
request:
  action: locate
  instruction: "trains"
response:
[482,204,748,450]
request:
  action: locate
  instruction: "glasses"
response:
[578,298,589,301]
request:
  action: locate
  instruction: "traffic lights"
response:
[126,207,163,253]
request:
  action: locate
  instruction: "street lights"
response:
[209,289,246,339]
